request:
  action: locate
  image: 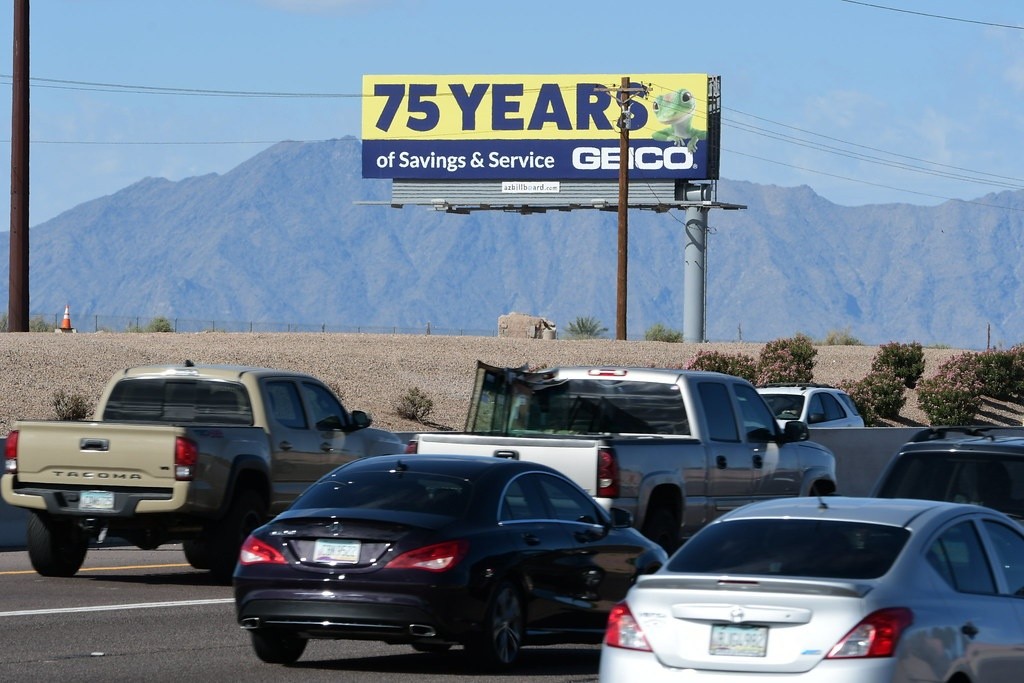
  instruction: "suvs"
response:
[874,424,1024,533]
[757,382,866,431]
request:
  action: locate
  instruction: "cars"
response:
[231,452,672,674]
[603,495,1024,682]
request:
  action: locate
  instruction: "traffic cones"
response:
[58,304,76,329]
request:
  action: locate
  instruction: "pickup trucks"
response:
[0,362,410,579]
[401,364,838,558]
